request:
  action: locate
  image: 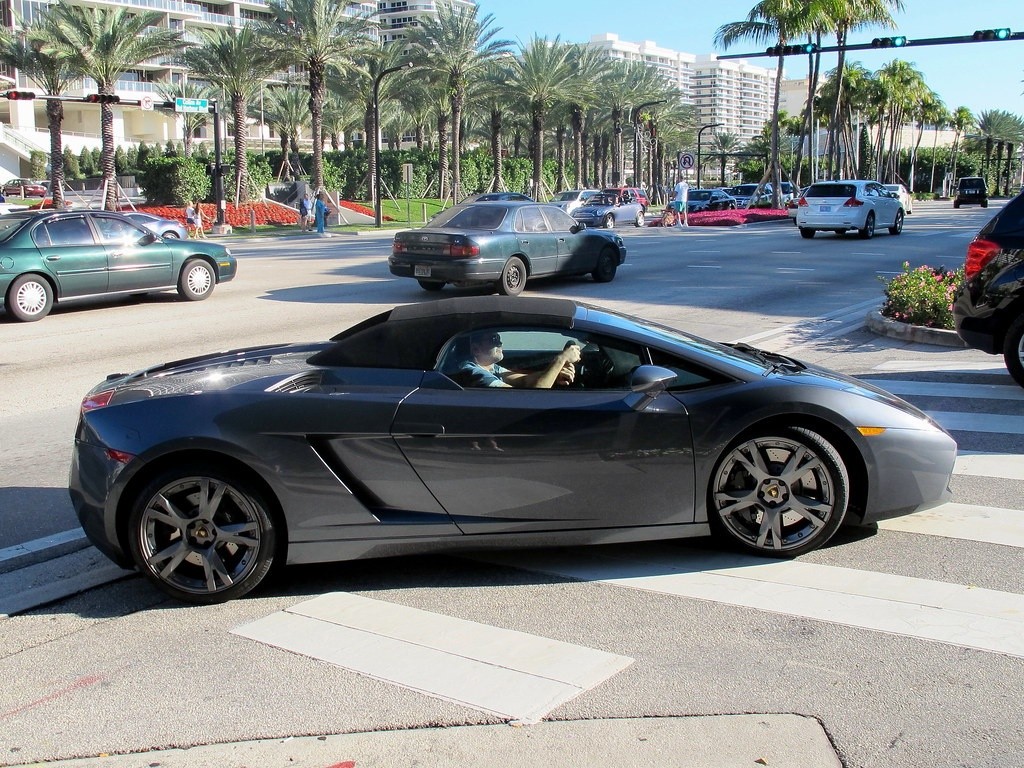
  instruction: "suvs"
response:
[594,188,649,214]
[952,190,1024,389]
[954,176,989,209]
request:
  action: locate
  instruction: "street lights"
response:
[697,123,725,190]
[634,100,668,187]
[374,61,414,228]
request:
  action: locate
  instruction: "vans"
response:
[549,190,600,215]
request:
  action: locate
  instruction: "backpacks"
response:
[300,207,307,216]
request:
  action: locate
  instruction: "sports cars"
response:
[68,296,959,605]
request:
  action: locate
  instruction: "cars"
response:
[570,194,645,229]
[788,180,912,239]
[1,178,47,198]
[388,193,626,295]
[0,209,237,323]
[727,183,796,210]
[666,190,737,216]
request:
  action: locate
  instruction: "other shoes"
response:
[675,223,683,228]
[682,223,689,227]
[188,232,194,237]
[201,233,207,239]
[195,234,200,239]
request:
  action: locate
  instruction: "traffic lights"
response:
[871,36,906,49]
[766,43,817,57]
[7,91,35,101]
[87,94,120,104]
[973,28,1011,42]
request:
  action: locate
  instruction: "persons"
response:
[457,330,581,388]
[192,203,209,239]
[602,194,619,205]
[316,194,325,233]
[673,176,689,227]
[301,194,313,233]
[186,200,201,239]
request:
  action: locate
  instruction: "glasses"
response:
[481,334,501,343]
